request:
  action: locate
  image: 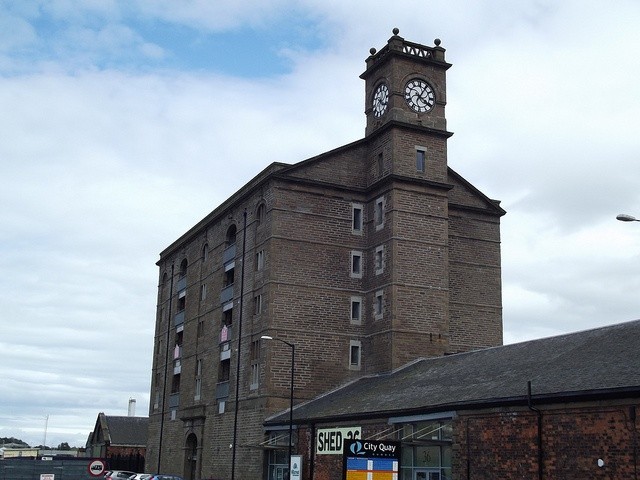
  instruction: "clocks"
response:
[371,84,390,118]
[404,79,435,114]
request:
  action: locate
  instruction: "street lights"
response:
[261,336,294,479]
